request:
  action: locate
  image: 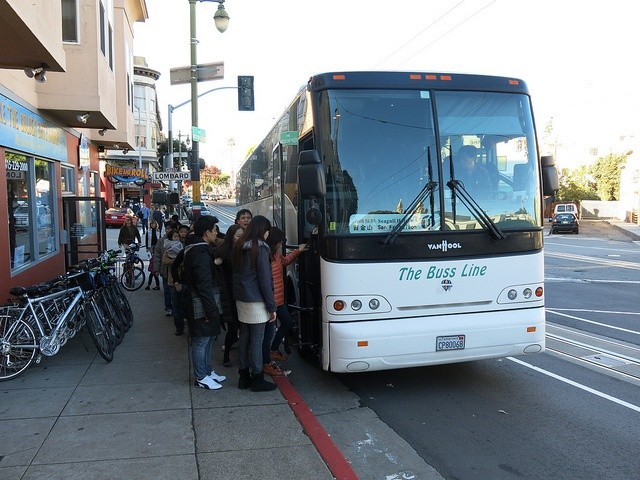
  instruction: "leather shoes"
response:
[224,361,232,367]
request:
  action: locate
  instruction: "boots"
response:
[250,370,277,391]
[238,369,252,389]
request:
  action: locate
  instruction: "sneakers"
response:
[195,375,223,390]
[264,362,282,375]
[152,287,160,290]
[270,351,288,361]
[145,286,150,290]
[175,328,184,336]
[209,371,226,383]
[165,309,173,315]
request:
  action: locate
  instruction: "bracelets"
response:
[173,282,179,286]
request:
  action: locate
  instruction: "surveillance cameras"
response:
[124,148,129,151]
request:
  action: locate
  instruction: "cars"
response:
[548,212,579,234]
[105,207,138,227]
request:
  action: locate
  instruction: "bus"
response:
[233,70,559,378]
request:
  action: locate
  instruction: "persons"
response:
[231,215,279,392]
[453,146,491,195]
[171,215,181,228]
[122,201,140,214]
[150,203,170,228]
[140,203,151,234]
[182,215,227,390]
[263,226,309,375]
[221,224,245,367]
[145,220,161,255]
[221,325,240,350]
[171,231,199,294]
[152,220,177,316]
[118,217,141,257]
[214,232,226,248]
[163,230,183,259]
[145,246,160,290]
[235,210,252,229]
[162,225,190,336]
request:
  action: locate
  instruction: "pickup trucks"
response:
[554,203,578,217]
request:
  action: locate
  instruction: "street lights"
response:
[187,0,230,216]
[177,130,190,203]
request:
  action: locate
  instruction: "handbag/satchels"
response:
[192,294,223,320]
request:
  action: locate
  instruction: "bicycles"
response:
[0,242,145,381]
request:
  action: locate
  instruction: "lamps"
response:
[35,71,47,83]
[76,113,90,124]
[24,66,41,78]
